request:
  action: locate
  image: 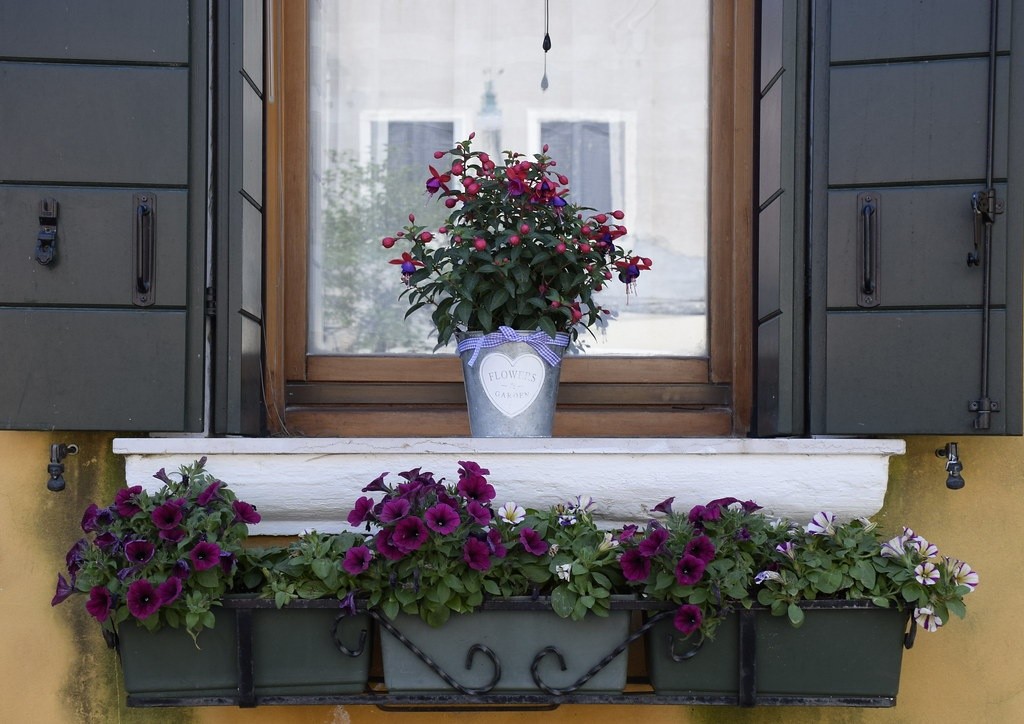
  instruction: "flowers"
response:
[618,491,980,641]
[341,456,629,630]
[381,132,653,341]
[47,458,359,648]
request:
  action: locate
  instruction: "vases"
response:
[382,593,634,695]
[114,589,373,702]
[455,331,571,440]
[639,589,917,699]
